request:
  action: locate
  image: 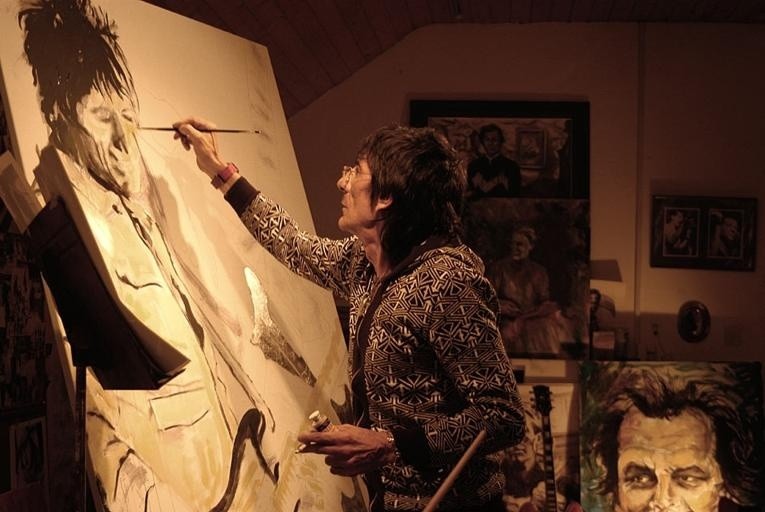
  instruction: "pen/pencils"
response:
[134,125,261,134]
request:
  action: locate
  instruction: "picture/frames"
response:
[406,97,588,358]
[649,194,759,273]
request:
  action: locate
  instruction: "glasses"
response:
[341,164,376,186]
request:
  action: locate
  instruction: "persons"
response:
[20,0,354,512]
[502,390,547,500]
[596,365,760,511]
[466,123,523,199]
[666,210,695,255]
[483,226,572,354]
[169,114,526,511]
[713,211,742,254]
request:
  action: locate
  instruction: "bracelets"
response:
[208,160,239,189]
[380,431,402,465]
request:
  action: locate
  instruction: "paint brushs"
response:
[420,430,488,512]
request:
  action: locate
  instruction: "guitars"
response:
[518,384,583,512]
